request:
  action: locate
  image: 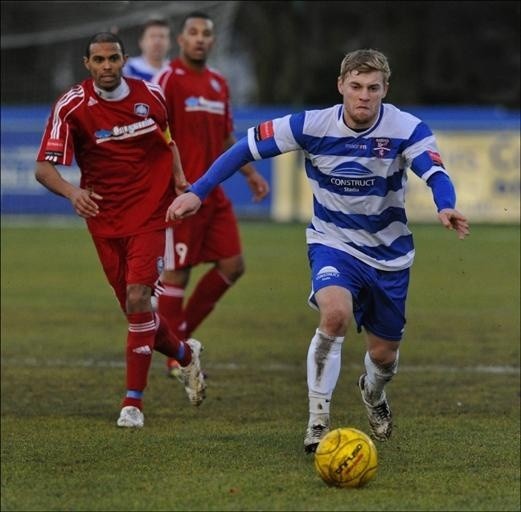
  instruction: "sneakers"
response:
[175,339,208,407]
[117,405,145,428]
[356,372,393,442]
[303,417,331,455]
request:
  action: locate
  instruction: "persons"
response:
[120,18,172,312]
[149,10,269,378]
[34,33,207,428]
[164,49,471,449]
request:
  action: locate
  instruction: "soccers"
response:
[315,428,377,488]
